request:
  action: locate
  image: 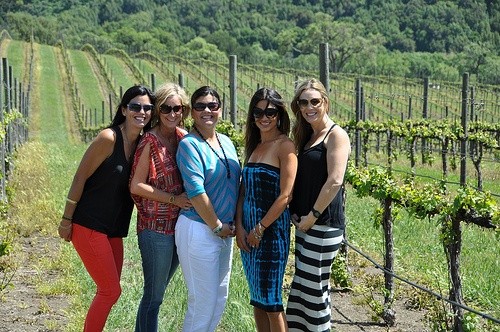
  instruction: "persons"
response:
[174,85,242,332]
[234,88,298,332]
[58,85,157,332]
[128,83,192,332]
[286,78,351,332]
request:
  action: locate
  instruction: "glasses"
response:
[253,108,279,119]
[192,102,221,112]
[127,103,155,112]
[159,104,187,114]
[298,98,324,107]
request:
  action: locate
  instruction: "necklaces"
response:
[125,131,136,157]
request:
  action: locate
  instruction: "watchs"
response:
[169,196,174,202]
[312,208,320,218]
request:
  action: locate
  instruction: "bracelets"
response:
[59,223,72,229]
[253,222,267,240]
[62,216,72,220]
[213,223,223,233]
[67,198,77,204]
